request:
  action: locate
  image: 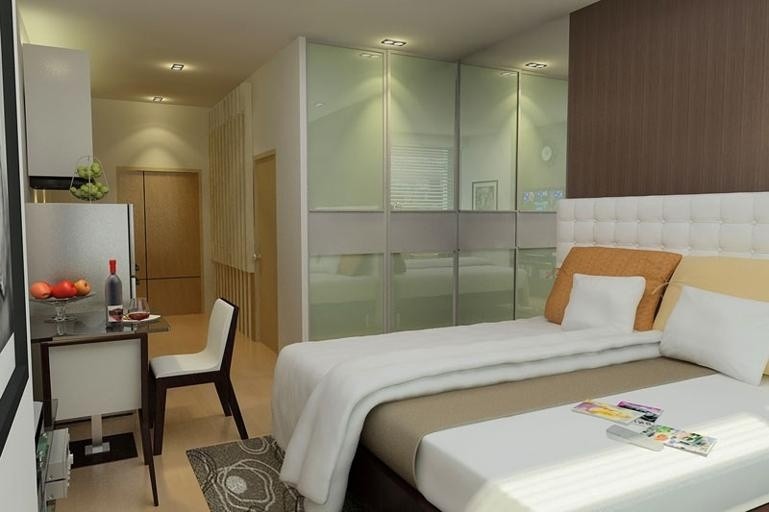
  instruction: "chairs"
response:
[42,334,162,506]
[148,296,246,452]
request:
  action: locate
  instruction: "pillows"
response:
[544,246,676,332]
[560,269,644,335]
[658,288,769,385]
[653,258,769,371]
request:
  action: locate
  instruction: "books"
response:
[615,400,664,427]
[638,423,719,457]
[572,399,647,425]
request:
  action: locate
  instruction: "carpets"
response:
[186,434,304,512]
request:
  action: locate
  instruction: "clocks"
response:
[540,142,556,166]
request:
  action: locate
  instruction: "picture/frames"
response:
[471,181,500,209]
[0,0,31,457]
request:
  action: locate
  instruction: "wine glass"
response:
[127,296,150,333]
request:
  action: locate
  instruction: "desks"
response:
[31,303,173,473]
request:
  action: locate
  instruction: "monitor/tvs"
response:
[516,188,564,212]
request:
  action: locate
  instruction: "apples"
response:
[31,281,52,299]
[51,279,77,298]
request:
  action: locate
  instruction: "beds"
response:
[267,193,769,510]
[308,256,527,342]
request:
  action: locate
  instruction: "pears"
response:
[74,279,90,296]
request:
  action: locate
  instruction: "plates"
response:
[121,312,161,325]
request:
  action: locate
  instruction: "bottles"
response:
[105,260,124,332]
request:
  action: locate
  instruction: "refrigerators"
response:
[25,201,141,402]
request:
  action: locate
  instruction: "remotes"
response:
[606,424,664,451]
